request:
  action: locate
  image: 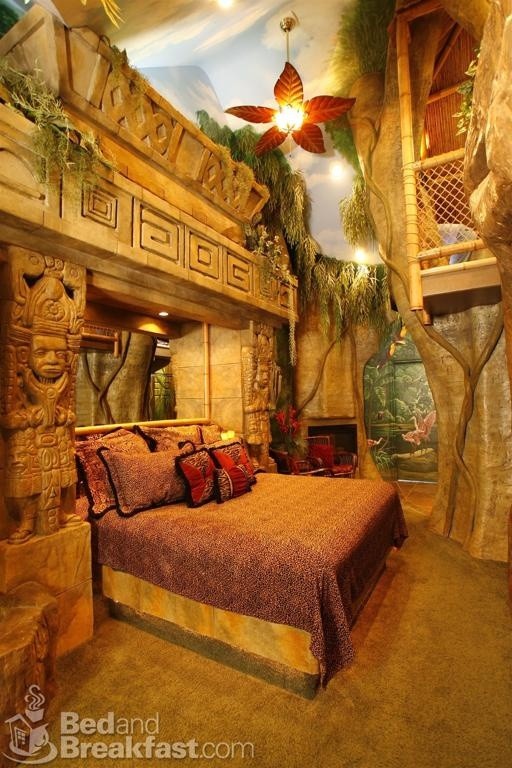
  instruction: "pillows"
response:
[74,422,257,517]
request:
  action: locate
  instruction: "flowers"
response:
[275,404,302,441]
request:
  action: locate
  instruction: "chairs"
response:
[268,436,357,477]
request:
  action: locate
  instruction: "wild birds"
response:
[367,437,385,447]
[400,407,436,458]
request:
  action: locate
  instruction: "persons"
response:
[1,243,87,545]
[242,319,277,467]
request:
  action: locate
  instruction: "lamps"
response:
[228,13,357,155]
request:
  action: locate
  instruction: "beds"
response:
[72,417,400,701]
